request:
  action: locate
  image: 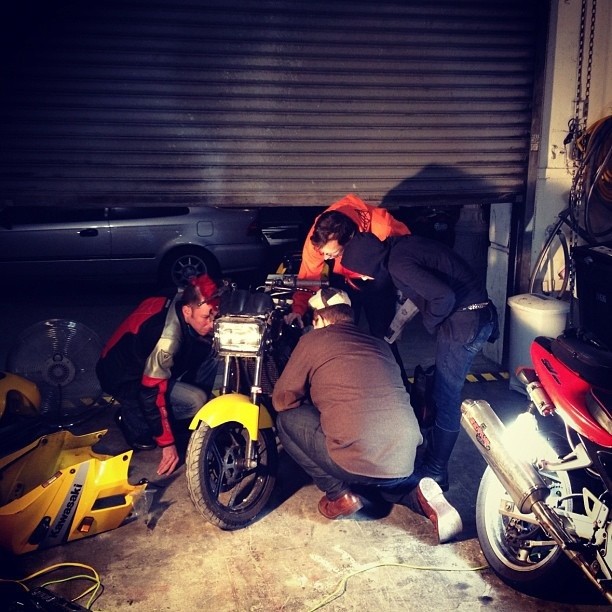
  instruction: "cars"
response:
[1,203,302,293]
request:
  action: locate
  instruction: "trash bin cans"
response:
[507,293,571,396]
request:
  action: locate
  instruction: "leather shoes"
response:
[318,490,364,519]
[416,477,463,544]
[114,410,156,450]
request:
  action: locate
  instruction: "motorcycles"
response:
[0,318,161,612]
[184,273,331,530]
[458,325,611,612]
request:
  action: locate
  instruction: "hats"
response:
[308,287,351,310]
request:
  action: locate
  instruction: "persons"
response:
[278,193,414,327]
[272,282,465,545]
[342,232,501,493]
[92,275,222,478]
